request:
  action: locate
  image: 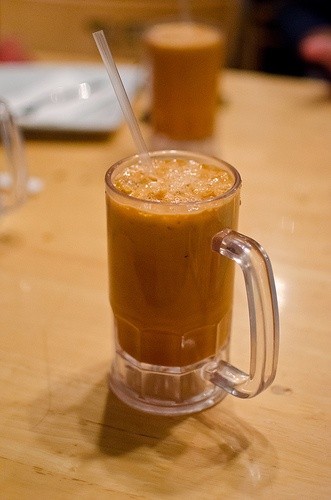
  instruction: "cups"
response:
[104,149,280,415]
[0,97,30,213]
[144,11,227,146]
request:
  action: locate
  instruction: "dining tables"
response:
[0,40,331,499]
